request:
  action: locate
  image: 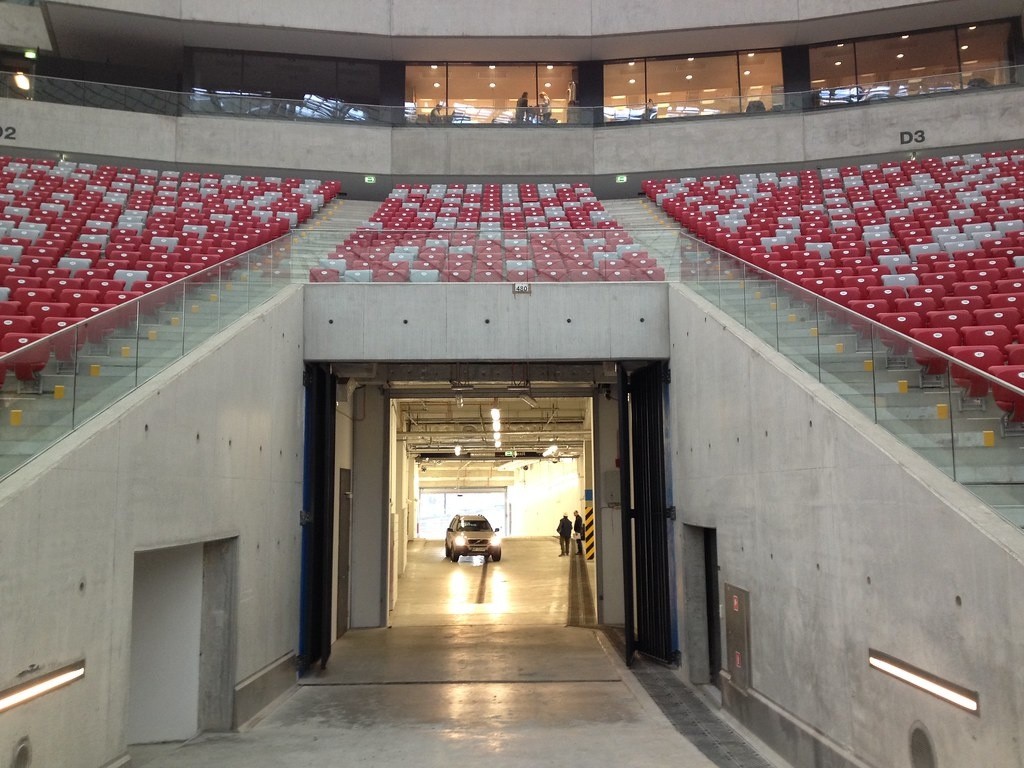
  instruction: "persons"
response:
[538,92,551,121]
[431,104,444,122]
[647,98,656,108]
[515,92,528,120]
[556,512,573,557]
[480,522,487,529]
[574,510,583,555]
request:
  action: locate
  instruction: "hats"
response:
[538,91,547,96]
[563,512,568,517]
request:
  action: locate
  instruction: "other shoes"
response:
[566,553,569,556]
[559,554,565,557]
[575,551,582,555]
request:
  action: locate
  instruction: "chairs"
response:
[640,150,1024,438]
[309,183,665,282]
[1,155,343,387]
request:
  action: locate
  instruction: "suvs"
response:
[444,514,502,562]
